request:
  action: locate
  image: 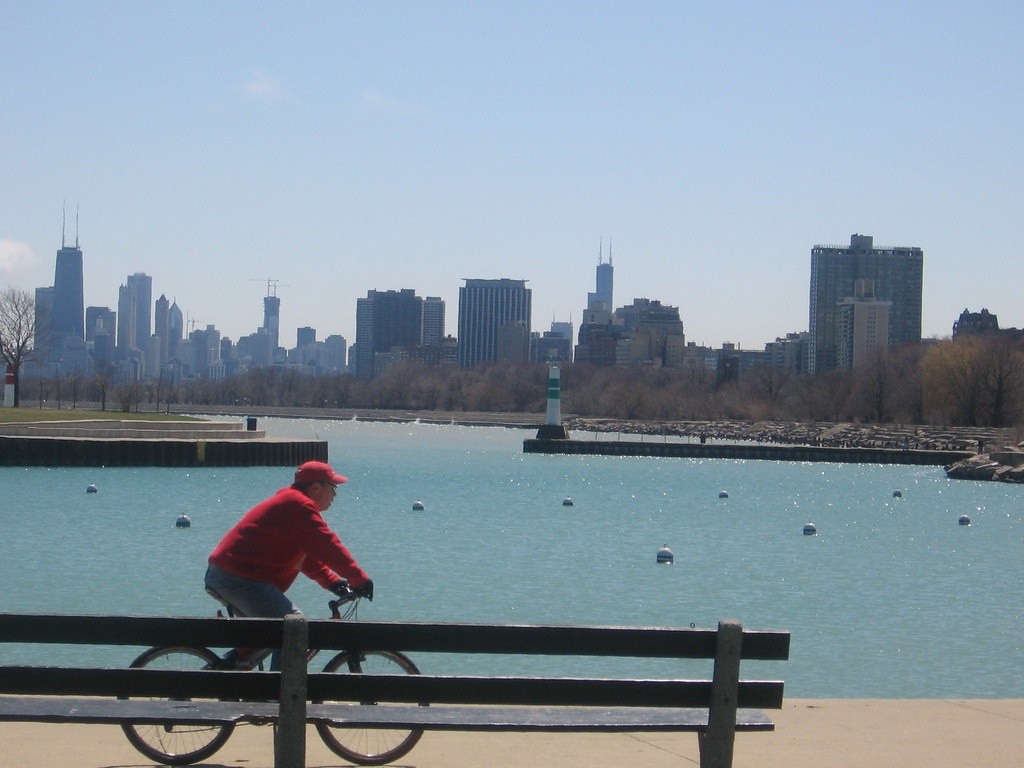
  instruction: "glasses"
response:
[323,480,338,491]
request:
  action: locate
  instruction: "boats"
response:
[943,452,999,480]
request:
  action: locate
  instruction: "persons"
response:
[205,461,373,671]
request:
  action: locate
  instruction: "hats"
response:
[295,461,348,484]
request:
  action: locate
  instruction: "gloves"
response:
[328,579,351,599]
[353,579,373,602]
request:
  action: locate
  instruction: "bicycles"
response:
[118,590,430,767]
